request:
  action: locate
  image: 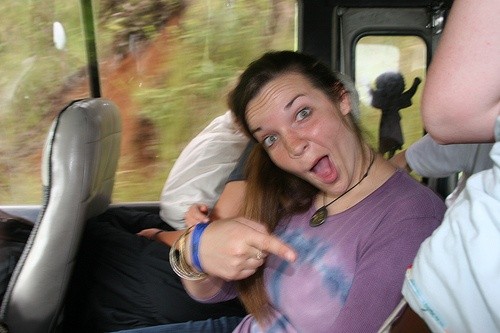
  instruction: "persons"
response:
[375,0,500,333]
[101,50,447,333]
[87,128,273,326]
[389,133,494,182]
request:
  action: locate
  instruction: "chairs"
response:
[0,95,124,333]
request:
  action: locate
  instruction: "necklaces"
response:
[307,146,375,228]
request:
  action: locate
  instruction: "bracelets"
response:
[168,226,208,281]
[192,219,210,275]
[179,224,195,276]
